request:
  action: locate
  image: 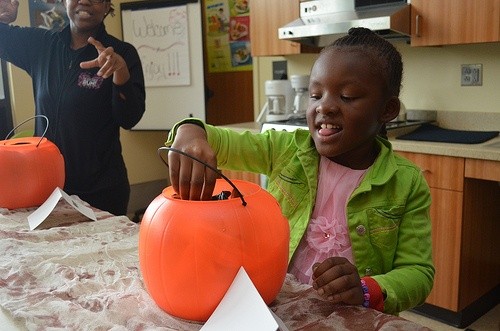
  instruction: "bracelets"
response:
[361,278,371,309]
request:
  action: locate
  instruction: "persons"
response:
[164,28,436,317]
[0,0,146,216]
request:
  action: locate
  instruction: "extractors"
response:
[278,0,411,48]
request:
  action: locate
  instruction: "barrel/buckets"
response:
[139,147,290,325]
[0,115,65,211]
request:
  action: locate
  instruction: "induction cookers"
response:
[260,114,440,142]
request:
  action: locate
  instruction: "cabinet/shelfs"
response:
[410,0,500,47]
[216,150,500,328]
[249,0,300,56]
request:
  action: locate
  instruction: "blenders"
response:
[289,74,311,118]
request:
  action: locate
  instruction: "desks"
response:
[0,194,431,331]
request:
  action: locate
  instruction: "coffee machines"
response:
[264,80,296,122]
